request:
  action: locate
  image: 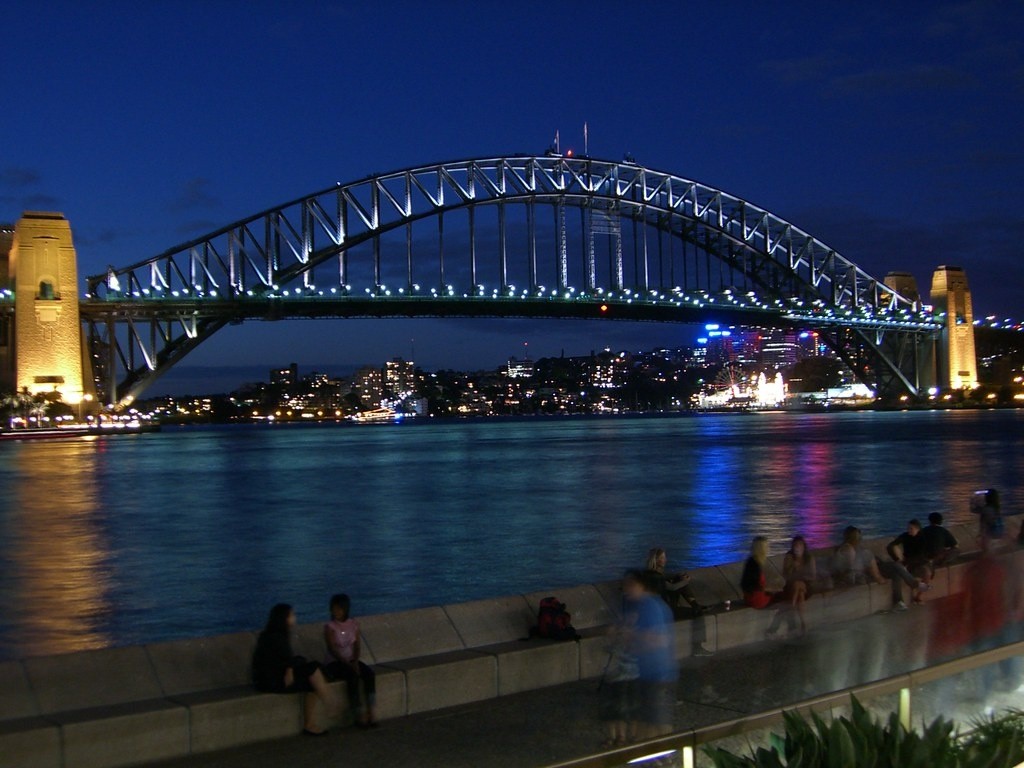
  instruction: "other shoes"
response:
[893,601,907,614]
[303,728,328,737]
[916,582,932,594]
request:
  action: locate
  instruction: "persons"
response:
[251,603,347,736]
[831,511,960,615]
[781,534,816,637]
[975,488,1005,561]
[323,593,377,728]
[741,536,801,640]
[604,548,716,747]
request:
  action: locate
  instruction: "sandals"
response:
[601,735,626,752]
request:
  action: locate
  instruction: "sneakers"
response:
[692,647,713,658]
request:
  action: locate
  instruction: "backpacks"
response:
[536,596,582,643]
